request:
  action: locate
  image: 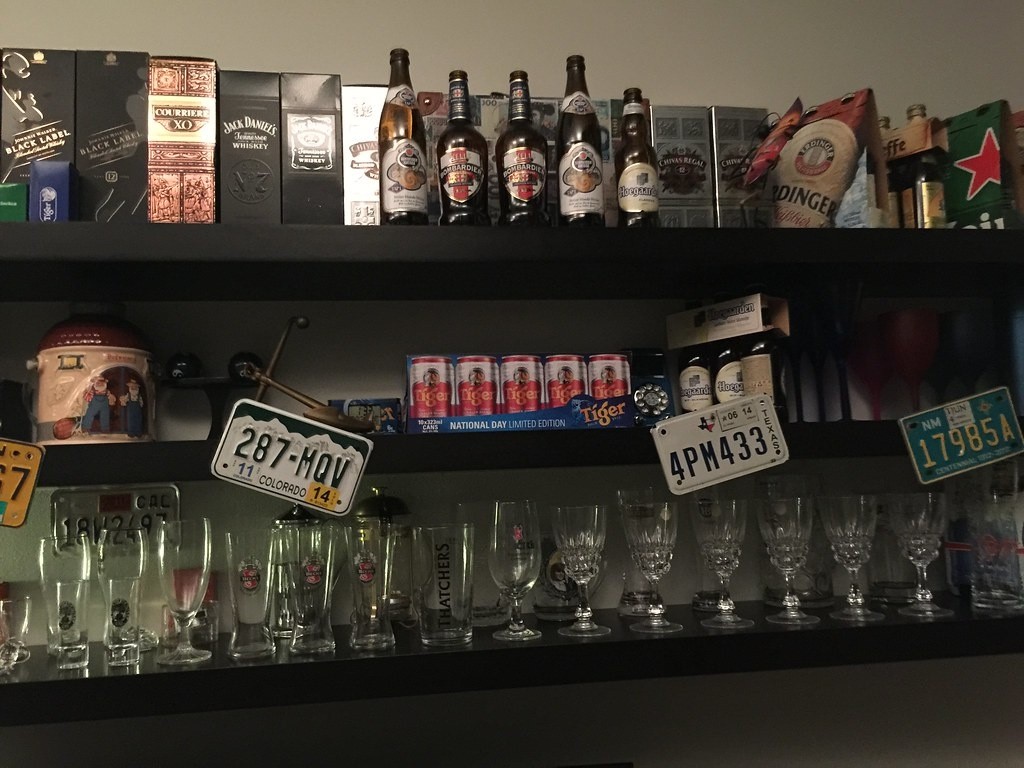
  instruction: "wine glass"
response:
[155,517,211,666]
[489,490,953,642]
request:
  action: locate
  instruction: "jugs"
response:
[258,488,351,638]
[345,487,434,629]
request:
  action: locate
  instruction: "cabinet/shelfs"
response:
[0,221,1024,728]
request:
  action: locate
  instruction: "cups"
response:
[108,577,141,666]
[95,526,146,650]
[617,487,666,617]
[690,486,733,611]
[225,522,472,659]
[858,473,918,602]
[37,532,91,654]
[0,597,30,671]
[530,530,607,622]
[755,471,835,607]
[448,500,512,628]
[969,492,1023,610]
[56,580,90,670]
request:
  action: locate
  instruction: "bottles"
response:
[495,70,552,228]
[436,69,491,227]
[616,87,660,230]
[556,54,606,230]
[678,260,788,425]
[378,47,429,227]
[875,104,948,229]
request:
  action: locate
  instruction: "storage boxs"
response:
[0,48,780,230]
